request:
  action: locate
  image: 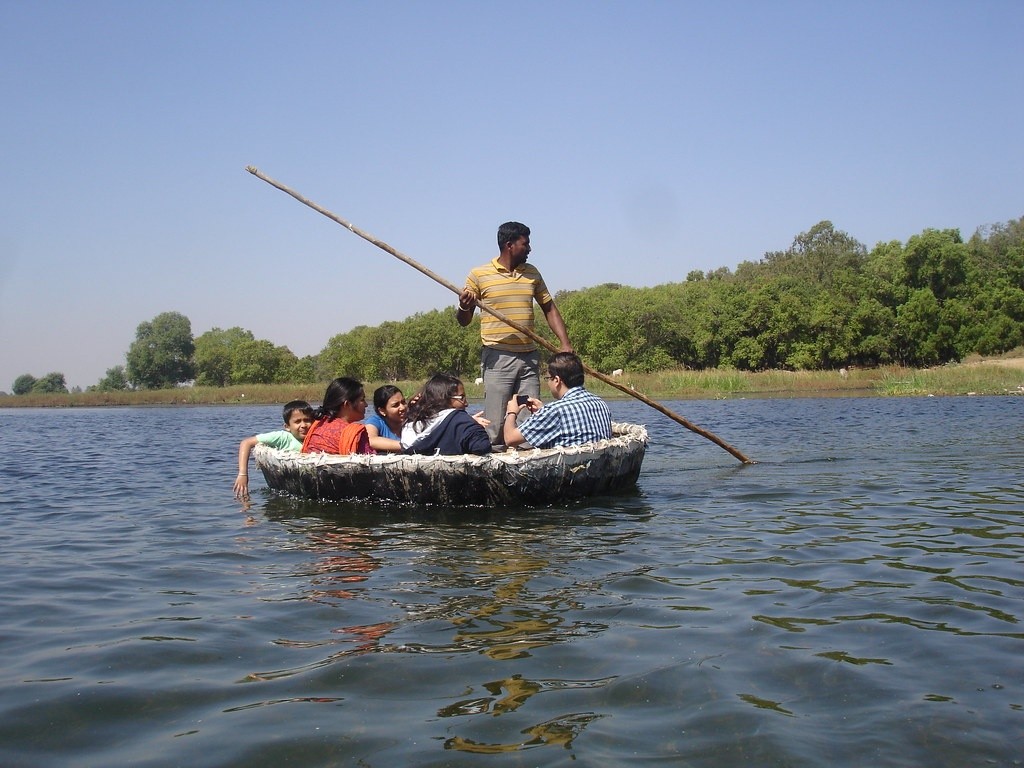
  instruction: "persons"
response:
[302,377,378,455]
[457,222,576,454]
[504,352,612,448]
[365,386,491,456]
[233,400,317,498]
[401,374,491,457]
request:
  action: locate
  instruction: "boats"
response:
[253,420,650,510]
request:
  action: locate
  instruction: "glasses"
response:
[544,375,562,382]
[451,393,466,403]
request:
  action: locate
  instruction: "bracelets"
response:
[504,412,517,420]
[238,474,248,476]
[458,303,470,311]
